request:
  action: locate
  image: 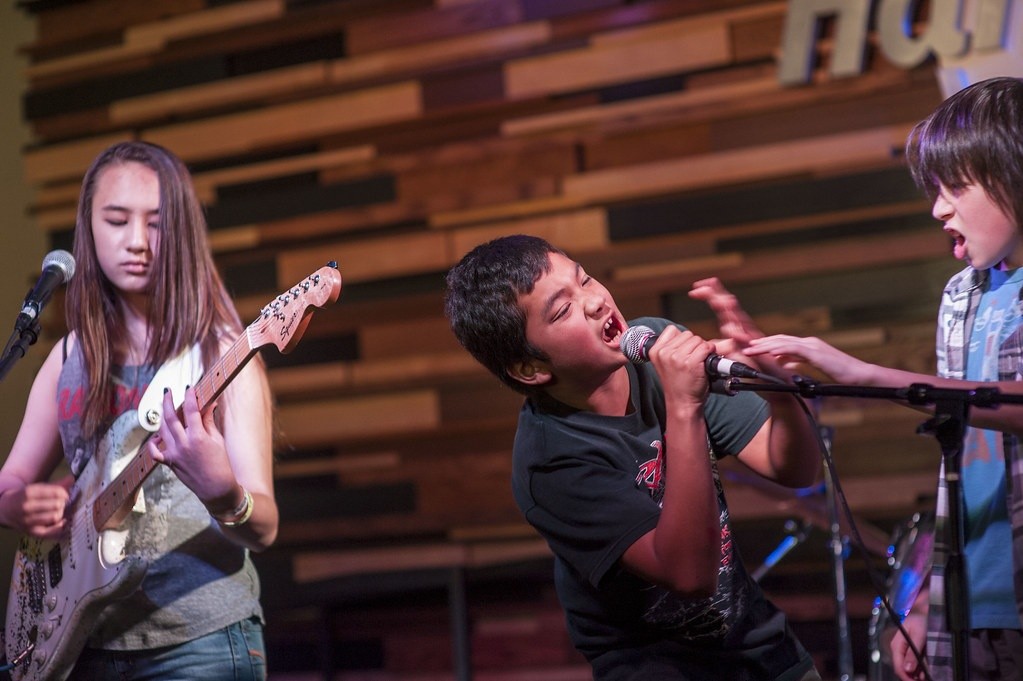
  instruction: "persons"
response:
[0,140,278,680]
[742,75,1023,681]
[442,235,827,681]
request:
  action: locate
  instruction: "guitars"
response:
[4,256,343,681]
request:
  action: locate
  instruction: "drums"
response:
[868,512,938,670]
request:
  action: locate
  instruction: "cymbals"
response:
[720,468,891,555]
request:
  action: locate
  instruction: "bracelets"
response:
[0,488,12,530]
[209,485,254,529]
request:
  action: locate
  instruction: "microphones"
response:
[13,250,76,334]
[619,326,759,379]
